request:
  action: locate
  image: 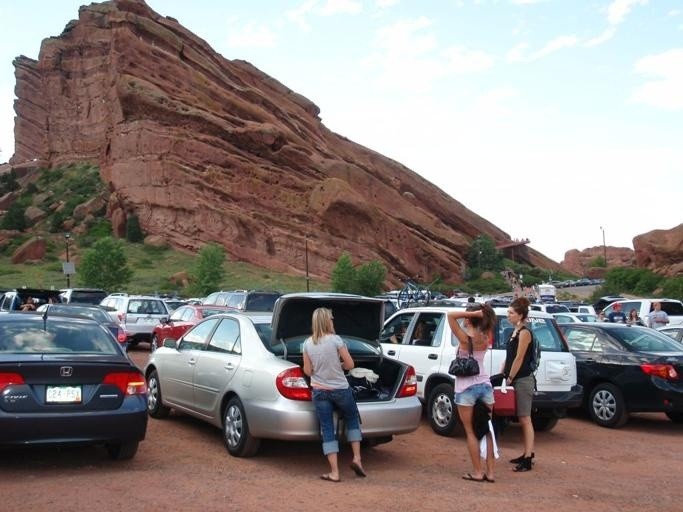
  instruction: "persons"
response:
[501,297,538,473]
[596,311,604,321]
[647,302,669,330]
[447,302,498,482]
[625,309,647,327]
[301,307,366,481]
[389,323,431,346]
[467,297,475,303]
[608,303,627,323]
[17,296,35,312]
[47,296,57,304]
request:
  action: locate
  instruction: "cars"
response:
[630,323,682,359]
[559,320,682,431]
[0,304,151,469]
[142,290,424,458]
[0,285,285,351]
[368,273,631,344]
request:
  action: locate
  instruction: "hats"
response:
[612,303,621,308]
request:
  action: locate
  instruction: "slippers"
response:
[483,474,495,483]
[320,473,341,482]
[350,462,366,476]
[462,473,484,481]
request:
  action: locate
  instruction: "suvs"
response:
[597,298,682,328]
[375,304,582,441]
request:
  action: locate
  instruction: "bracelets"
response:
[508,375,512,381]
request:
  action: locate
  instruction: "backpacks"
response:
[519,324,542,392]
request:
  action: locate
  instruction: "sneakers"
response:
[513,457,531,472]
[509,452,534,463]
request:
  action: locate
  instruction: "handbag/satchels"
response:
[449,336,478,377]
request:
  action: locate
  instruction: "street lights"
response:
[598,226,607,267]
[61,232,73,288]
